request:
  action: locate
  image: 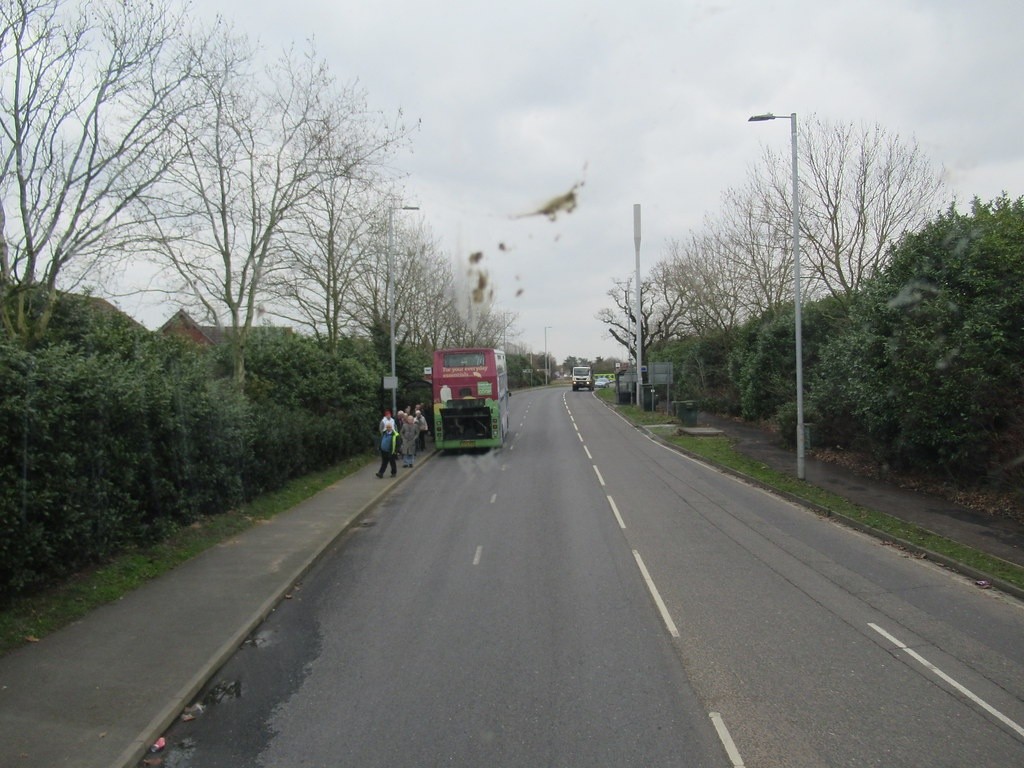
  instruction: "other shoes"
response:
[391,473,396,478]
[376,472,383,478]
[403,464,408,468]
[410,464,413,468]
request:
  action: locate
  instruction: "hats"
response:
[385,411,391,417]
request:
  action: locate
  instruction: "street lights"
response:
[748,111,806,481]
[503,311,513,353]
[544,326,552,385]
[389,207,420,424]
[616,281,634,407]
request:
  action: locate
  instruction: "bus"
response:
[433,347,508,450]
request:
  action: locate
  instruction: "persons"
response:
[400,416,420,468]
[395,404,428,459]
[376,423,402,478]
[379,409,397,437]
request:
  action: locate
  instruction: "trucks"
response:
[572,367,594,390]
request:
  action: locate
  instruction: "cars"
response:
[595,378,610,387]
